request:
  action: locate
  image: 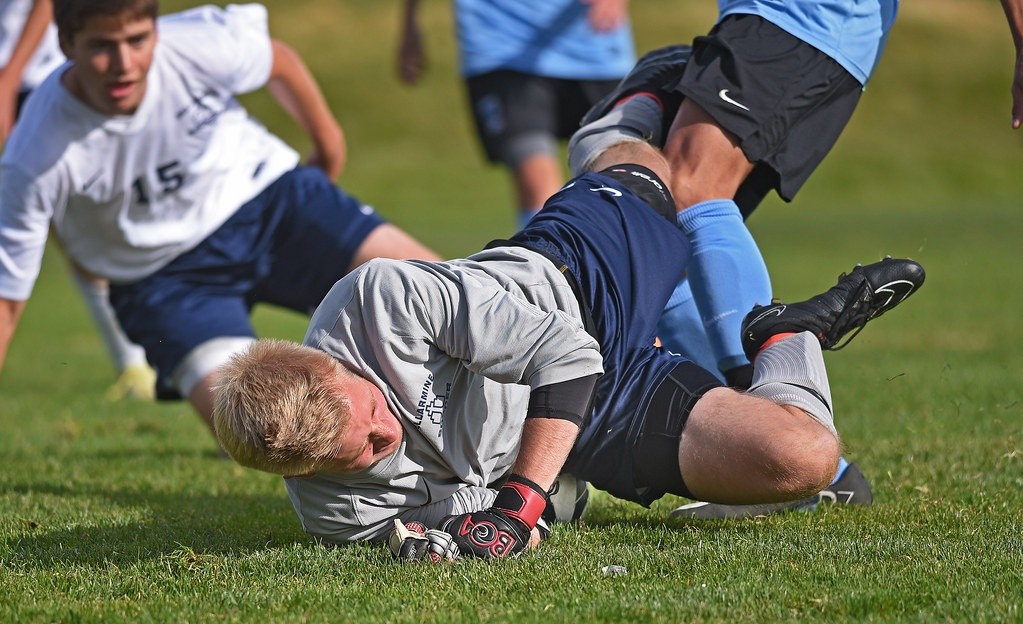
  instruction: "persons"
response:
[382,0,641,236]
[211,43,927,564]
[655,0,1023,528]
[1,0,445,428]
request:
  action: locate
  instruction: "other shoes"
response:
[105,361,157,405]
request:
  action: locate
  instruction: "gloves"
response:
[440,474,550,564]
[389,517,461,562]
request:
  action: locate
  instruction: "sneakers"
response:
[740,254,925,364]
[580,45,695,127]
[667,460,872,528]
